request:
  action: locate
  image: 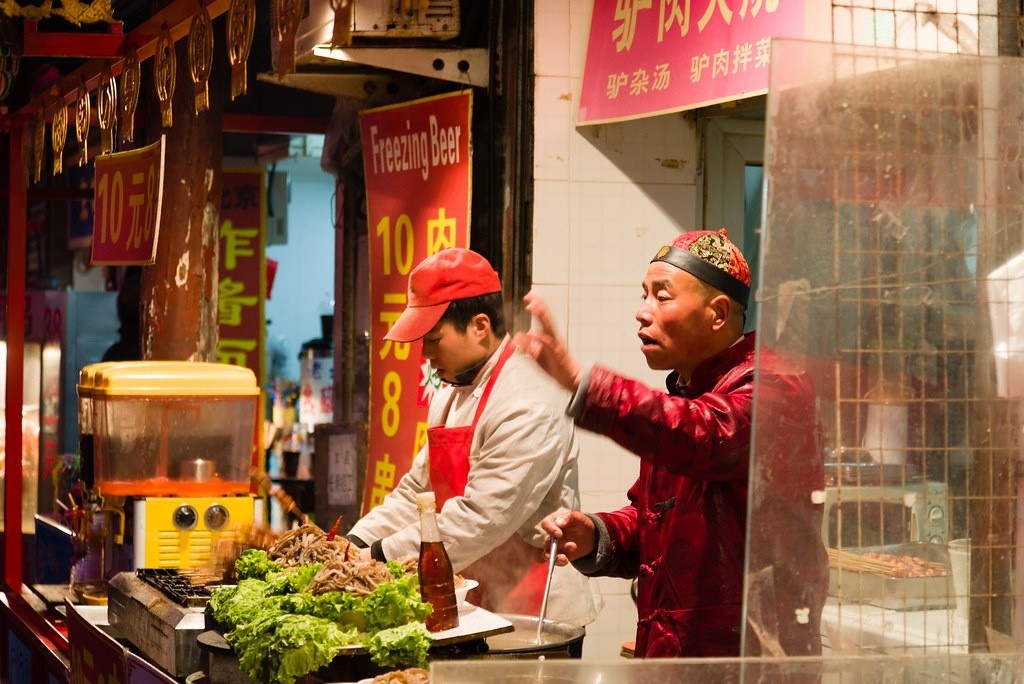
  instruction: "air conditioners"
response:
[292,0,462,68]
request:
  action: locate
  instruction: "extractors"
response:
[257,0,490,101]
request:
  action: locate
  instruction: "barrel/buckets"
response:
[950,537,971,622]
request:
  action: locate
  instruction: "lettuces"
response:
[205,547,435,684]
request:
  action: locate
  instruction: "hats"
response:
[383,247,503,343]
[650,229,752,309]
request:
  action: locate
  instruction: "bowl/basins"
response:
[74,586,108,606]
[83,592,108,606]
[417,579,479,611]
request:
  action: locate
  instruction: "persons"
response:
[342,246,604,658]
[511,228,831,684]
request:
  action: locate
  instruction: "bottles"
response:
[85,522,104,582]
[418,492,458,632]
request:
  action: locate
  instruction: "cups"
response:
[292,423,308,457]
[272,405,293,438]
[70,565,83,596]
[282,451,299,478]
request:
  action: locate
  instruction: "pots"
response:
[482,614,584,651]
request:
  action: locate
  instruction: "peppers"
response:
[327,516,343,542]
[344,537,351,561]
